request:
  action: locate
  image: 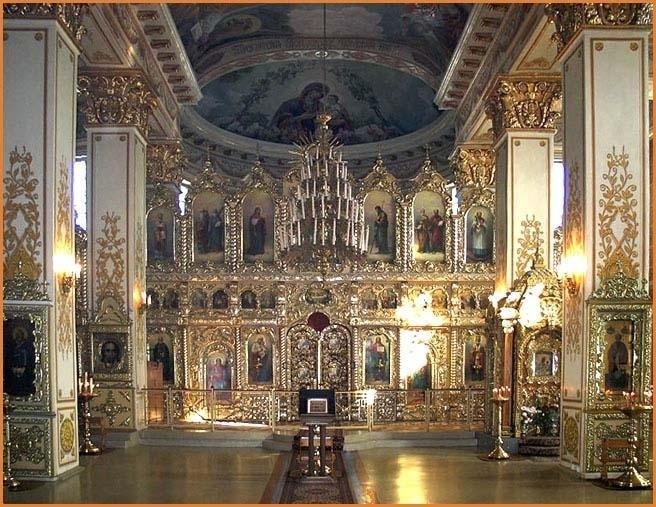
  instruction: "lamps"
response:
[273,4,370,273]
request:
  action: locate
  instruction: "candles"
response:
[492,385,511,399]
[76,264,80,278]
[78,369,93,394]
[143,294,151,304]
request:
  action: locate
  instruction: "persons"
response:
[609,330,628,386]
[98,340,120,360]
[267,79,346,145]
[8,323,29,391]
[325,94,350,121]
